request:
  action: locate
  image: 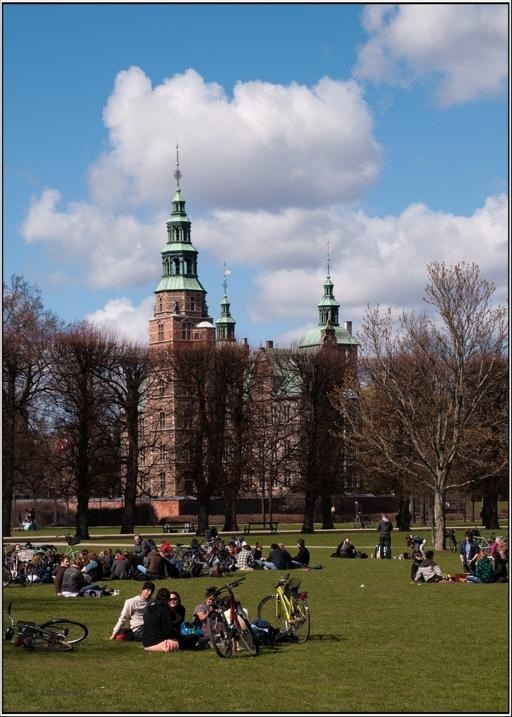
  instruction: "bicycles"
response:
[3,599,89,652]
[256,571,312,645]
[165,532,240,581]
[470,528,509,556]
[352,514,376,529]
[3,541,36,590]
[37,531,90,572]
[202,572,259,659]
[442,523,460,553]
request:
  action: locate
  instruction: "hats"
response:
[143,582,155,592]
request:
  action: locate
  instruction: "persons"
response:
[335,505,509,583]
[9,508,310,652]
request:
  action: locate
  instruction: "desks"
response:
[160,522,190,533]
[248,522,279,534]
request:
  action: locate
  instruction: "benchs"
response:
[244,526,280,535]
[164,527,189,533]
